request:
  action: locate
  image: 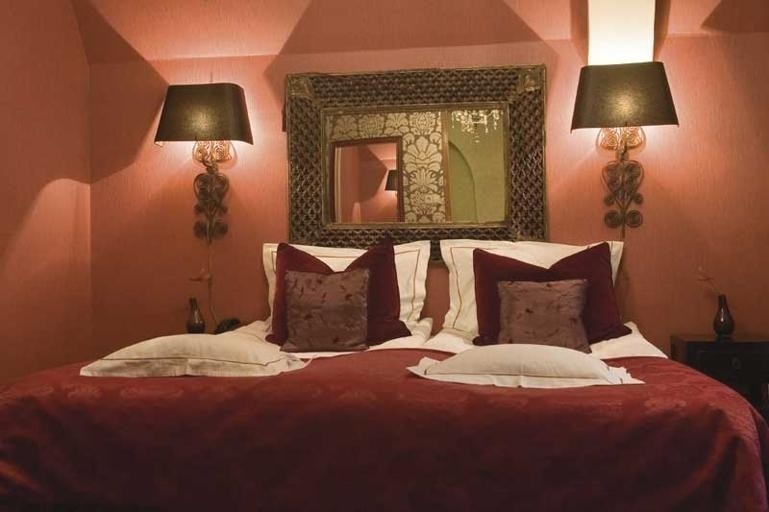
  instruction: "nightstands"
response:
[667,333,769,424]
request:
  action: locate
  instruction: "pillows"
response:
[470,243,631,347]
[439,237,627,336]
[79,332,309,378]
[279,271,368,352]
[488,278,592,356]
[263,242,432,340]
[269,243,413,344]
[406,343,645,388]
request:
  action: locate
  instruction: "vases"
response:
[713,294,736,338]
[185,296,206,334]
[689,260,723,297]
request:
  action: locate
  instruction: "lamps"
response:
[153,83,255,246]
[446,107,507,149]
[567,60,681,241]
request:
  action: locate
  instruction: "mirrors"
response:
[286,63,550,248]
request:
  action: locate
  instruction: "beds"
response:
[2,239,769,512]
